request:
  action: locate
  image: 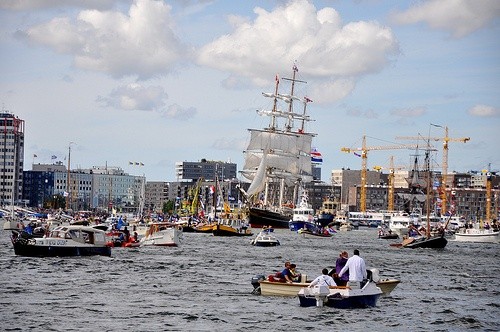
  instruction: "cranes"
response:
[371,156,448,213]
[395,126,471,216]
[472,163,500,224]
[339,133,434,212]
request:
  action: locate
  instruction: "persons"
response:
[308,269,337,288]
[281,262,301,282]
[289,264,299,281]
[336,252,349,286]
[338,249,368,288]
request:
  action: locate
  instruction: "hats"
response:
[289,264,296,268]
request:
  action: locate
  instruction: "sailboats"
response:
[236,63,334,229]
[401,157,449,250]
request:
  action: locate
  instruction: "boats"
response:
[298,267,385,309]
[455,220,500,244]
[250,274,401,297]
[0,193,355,258]
[377,214,468,240]
